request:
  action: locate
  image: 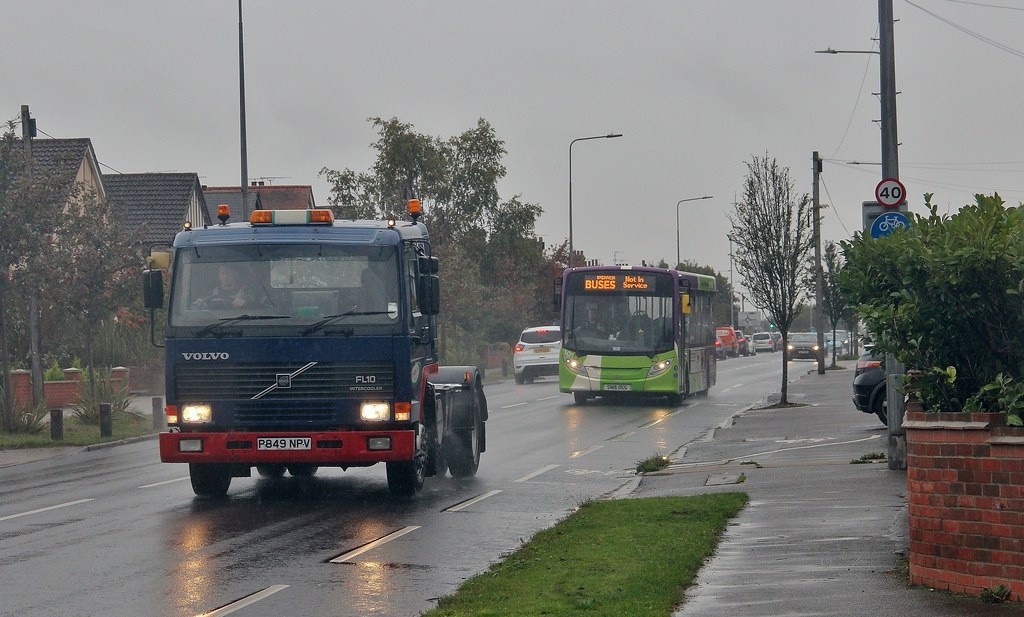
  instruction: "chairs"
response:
[632,311,648,320]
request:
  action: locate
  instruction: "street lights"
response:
[569,133,622,268]
[676,195,714,266]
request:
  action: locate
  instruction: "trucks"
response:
[143,198,490,502]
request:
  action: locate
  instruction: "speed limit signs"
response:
[875,177,907,208]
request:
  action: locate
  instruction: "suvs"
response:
[715,325,750,358]
[512,326,575,385]
[851,341,928,427]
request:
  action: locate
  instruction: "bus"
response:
[553,266,719,403]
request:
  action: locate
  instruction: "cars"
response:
[745,332,789,356]
[715,335,727,359]
[788,329,864,362]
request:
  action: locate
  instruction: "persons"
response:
[193,264,253,307]
[570,307,608,334]
